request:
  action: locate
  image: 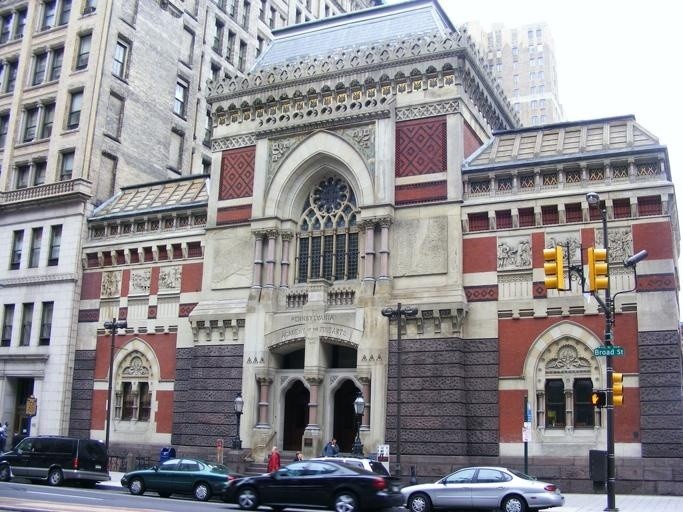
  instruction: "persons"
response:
[0,420,21,453]
[596,229,633,262]
[497,238,532,269]
[324,438,339,457]
[267,446,280,472]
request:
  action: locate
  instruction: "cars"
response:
[220,456,406,512]
[400,465,566,512]
[121,456,245,501]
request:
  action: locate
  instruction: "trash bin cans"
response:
[160,447,176,465]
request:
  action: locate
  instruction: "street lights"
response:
[382,303,419,478]
[586,193,617,512]
[233,392,245,451]
[104,318,128,450]
[353,392,366,459]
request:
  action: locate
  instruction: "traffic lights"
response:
[588,247,609,291]
[543,245,565,289]
[590,371,624,407]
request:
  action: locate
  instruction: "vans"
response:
[1,435,111,487]
